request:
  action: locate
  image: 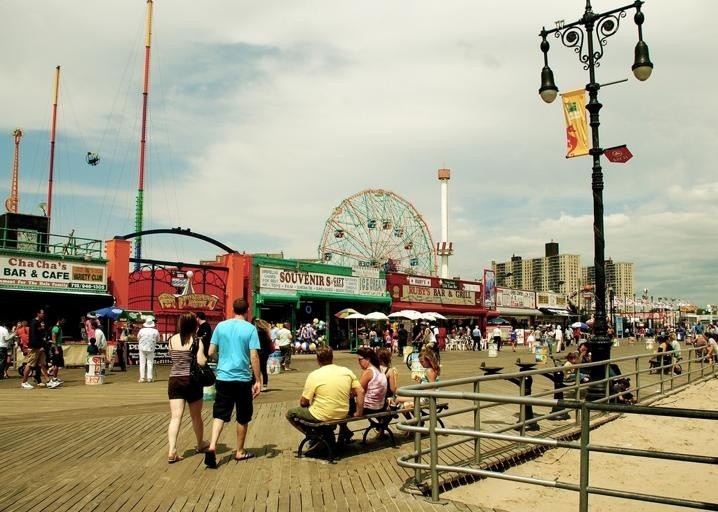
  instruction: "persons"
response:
[563,343,636,406]
[114,327,127,372]
[394,348,441,440]
[694,320,718,363]
[85,313,101,344]
[0,307,66,390]
[90,322,108,355]
[652,333,683,372]
[368,319,441,357]
[204,297,261,468]
[86,337,100,354]
[137,318,160,384]
[374,347,400,430]
[285,344,366,460]
[451,323,581,353]
[337,346,389,439]
[194,311,213,365]
[166,310,212,463]
[608,319,694,343]
[248,318,294,394]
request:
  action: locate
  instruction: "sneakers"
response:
[147,377,155,382]
[138,377,145,383]
[37,378,62,388]
[21,381,34,388]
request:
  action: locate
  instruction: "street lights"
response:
[536,4,654,401]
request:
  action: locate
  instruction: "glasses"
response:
[358,358,364,361]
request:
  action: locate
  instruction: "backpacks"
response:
[46,324,61,340]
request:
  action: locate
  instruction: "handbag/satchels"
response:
[189,357,216,386]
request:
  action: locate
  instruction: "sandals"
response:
[168,454,184,462]
[195,439,210,453]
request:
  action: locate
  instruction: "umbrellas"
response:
[343,314,367,354]
[333,308,360,339]
[367,309,449,322]
[568,321,590,329]
[93,306,125,319]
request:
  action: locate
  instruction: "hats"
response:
[143,318,157,327]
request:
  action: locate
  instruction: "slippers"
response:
[345,439,356,444]
[304,439,320,455]
[234,450,254,461]
[205,450,216,468]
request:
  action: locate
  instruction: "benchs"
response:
[293,401,449,463]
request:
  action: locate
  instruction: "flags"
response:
[559,88,591,159]
